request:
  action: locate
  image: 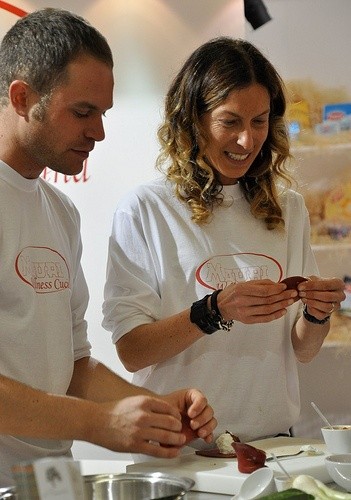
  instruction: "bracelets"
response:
[302,303,332,325]
[190,290,234,335]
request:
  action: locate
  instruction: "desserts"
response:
[195,429,267,473]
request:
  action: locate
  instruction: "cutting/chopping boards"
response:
[126,436,335,496]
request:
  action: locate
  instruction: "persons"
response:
[0,8,218,488]
[101,36,347,450]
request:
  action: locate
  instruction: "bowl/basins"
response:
[82,473,196,500]
[321,425,351,454]
[325,453,351,492]
[230,467,277,500]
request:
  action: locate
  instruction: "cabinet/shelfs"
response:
[293,144,351,348]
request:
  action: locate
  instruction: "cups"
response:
[274,475,295,492]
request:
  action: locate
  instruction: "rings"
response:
[329,303,335,314]
